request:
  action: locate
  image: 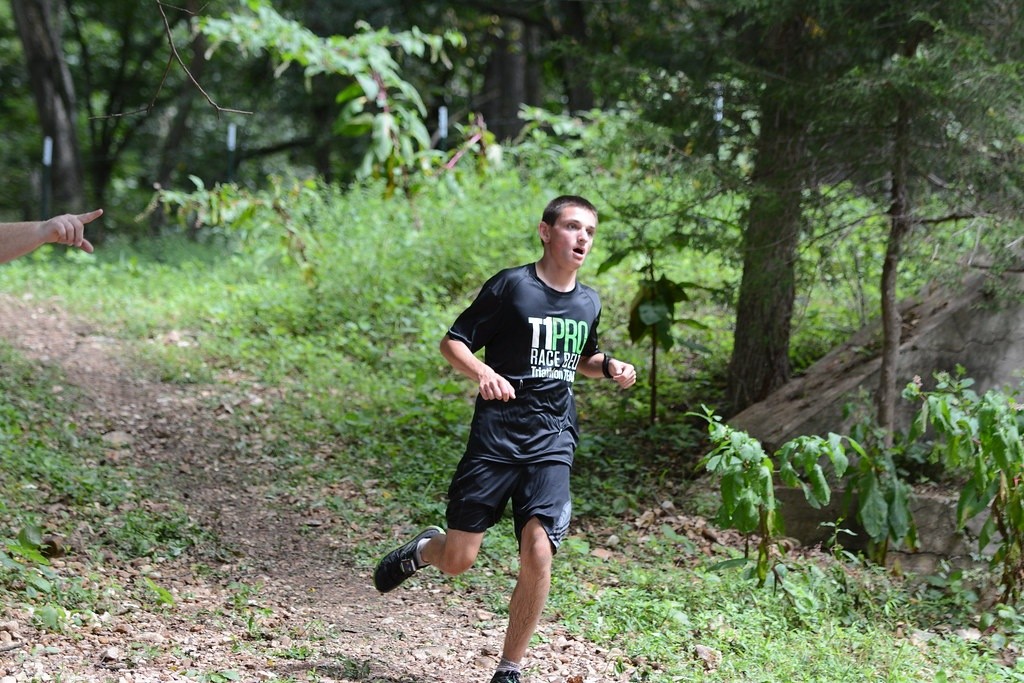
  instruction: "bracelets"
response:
[601,352,613,378]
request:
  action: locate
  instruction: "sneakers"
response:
[490,670,521,683]
[373,526,447,593]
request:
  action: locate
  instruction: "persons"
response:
[373,194,637,682]
[0,208,104,265]
